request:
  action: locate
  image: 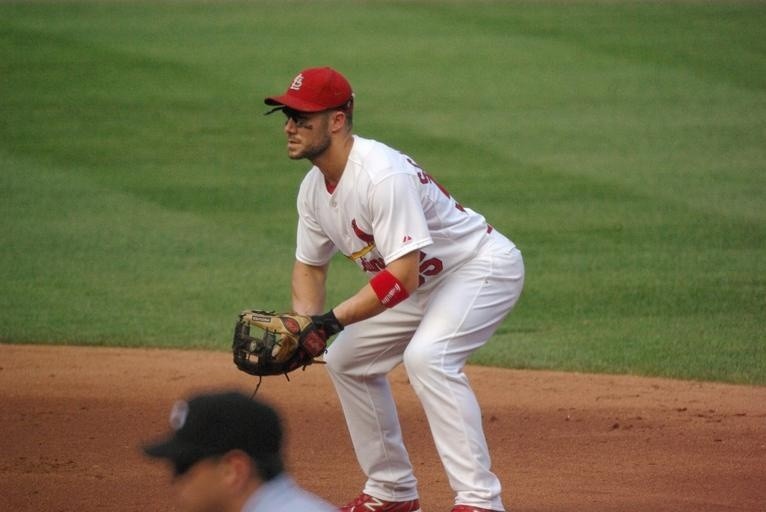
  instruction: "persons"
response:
[142,389,340,512]
[233,67,524,512]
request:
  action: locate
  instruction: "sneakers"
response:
[338,492,423,511]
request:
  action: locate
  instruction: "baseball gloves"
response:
[231,309,327,376]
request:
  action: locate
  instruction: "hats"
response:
[146,389,282,462]
[264,66,353,113]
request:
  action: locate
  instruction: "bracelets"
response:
[370,267,410,309]
